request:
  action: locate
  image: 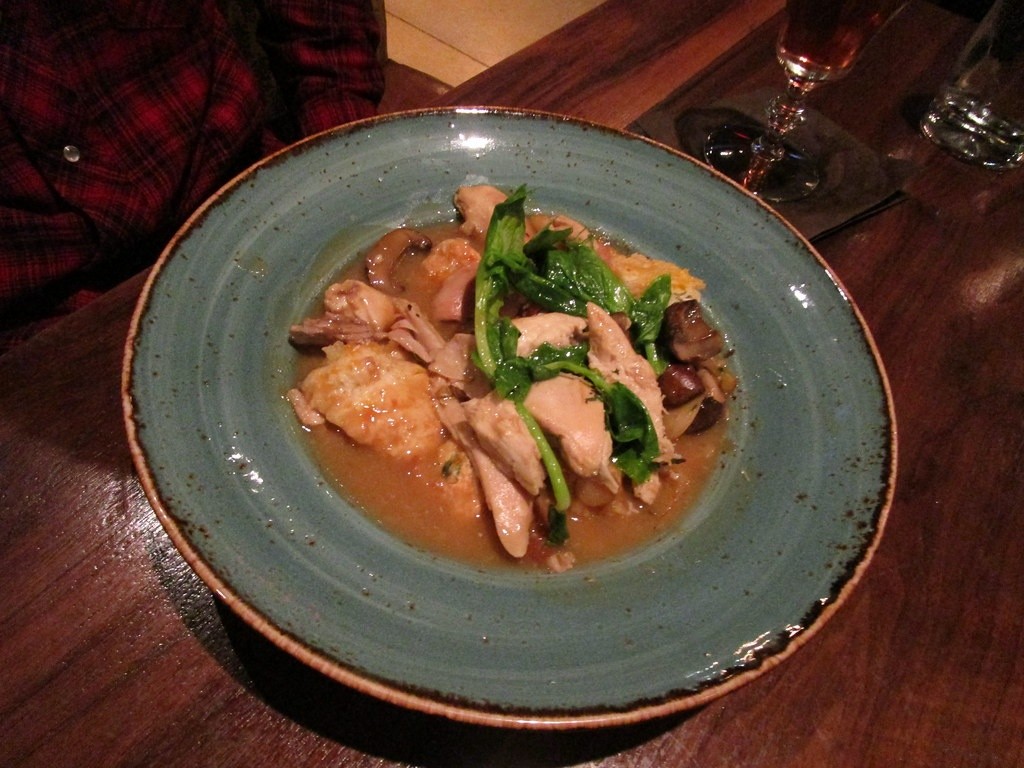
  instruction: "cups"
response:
[919,0,1024,170]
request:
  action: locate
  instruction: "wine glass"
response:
[703,0,911,206]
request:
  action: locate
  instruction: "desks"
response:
[0,0,1024,768]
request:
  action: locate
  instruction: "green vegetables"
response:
[472,182,674,546]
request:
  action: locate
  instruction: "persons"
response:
[1,1,386,341]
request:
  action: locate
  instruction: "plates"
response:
[123,107,897,730]
